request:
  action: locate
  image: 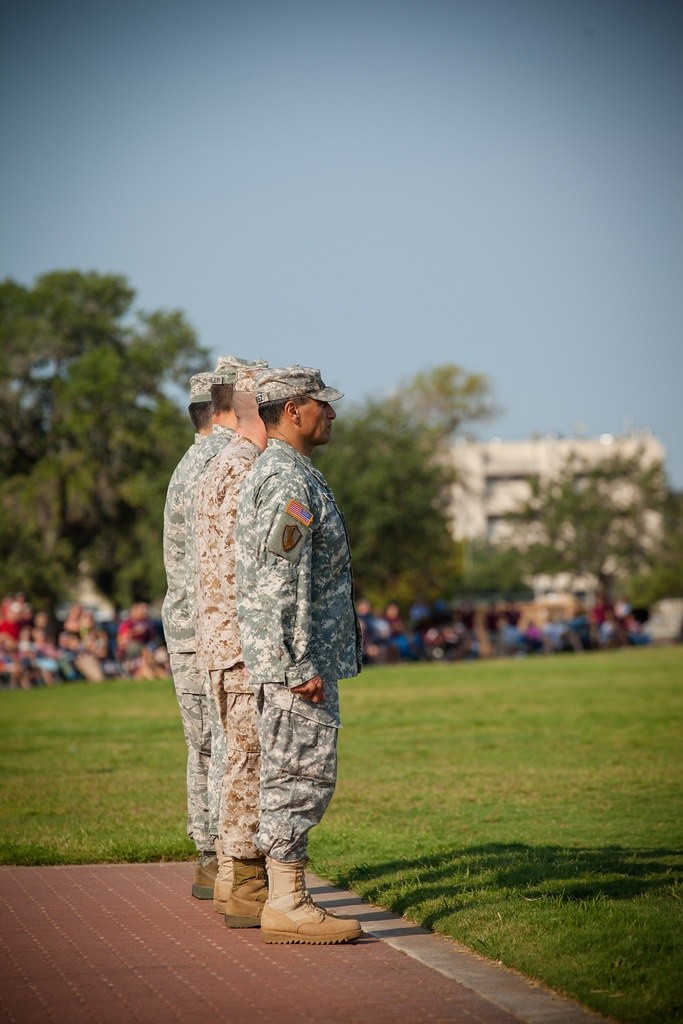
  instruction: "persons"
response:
[0,593,172,689]
[164,372,217,898]
[184,356,267,914]
[357,601,480,665]
[198,365,267,928]
[493,598,649,657]
[236,367,364,943]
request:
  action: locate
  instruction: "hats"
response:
[211,356,269,384]
[252,365,345,405]
[232,361,274,392]
[190,372,217,402]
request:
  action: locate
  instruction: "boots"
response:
[191,852,219,899]
[224,856,271,929]
[213,853,235,914]
[260,853,363,945]
